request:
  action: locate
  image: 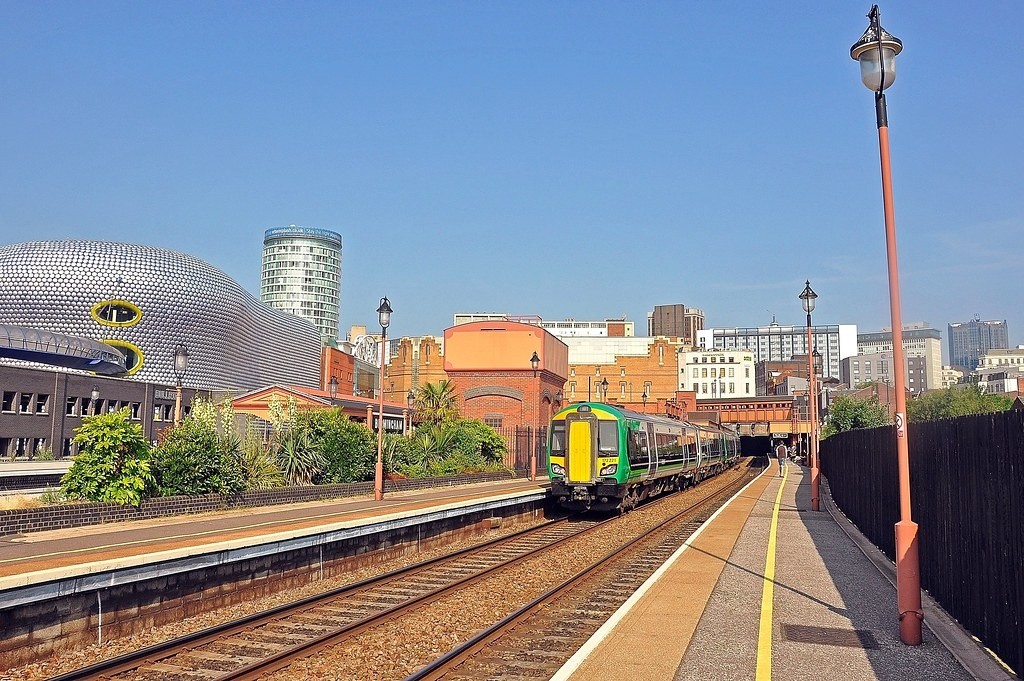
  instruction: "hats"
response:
[779,440,784,445]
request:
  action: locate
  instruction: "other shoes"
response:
[780,476,784,478]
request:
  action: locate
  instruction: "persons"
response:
[788,445,797,461]
[774,445,777,456]
[776,441,787,478]
[800,450,807,465]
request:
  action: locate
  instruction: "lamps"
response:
[555,390,563,407]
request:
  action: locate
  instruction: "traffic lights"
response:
[752,424,755,436]
[736,423,740,437]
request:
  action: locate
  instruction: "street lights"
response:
[328,376,339,409]
[800,279,818,514]
[406,392,416,442]
[374,296,394,501]
[641,392,647,414]
[172,342,190,429]
[809,346,822,486]
[803,390,810,469]
[529,352,541,482]
[601,377,609,403]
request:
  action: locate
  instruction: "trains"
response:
[544,403,741,518]
